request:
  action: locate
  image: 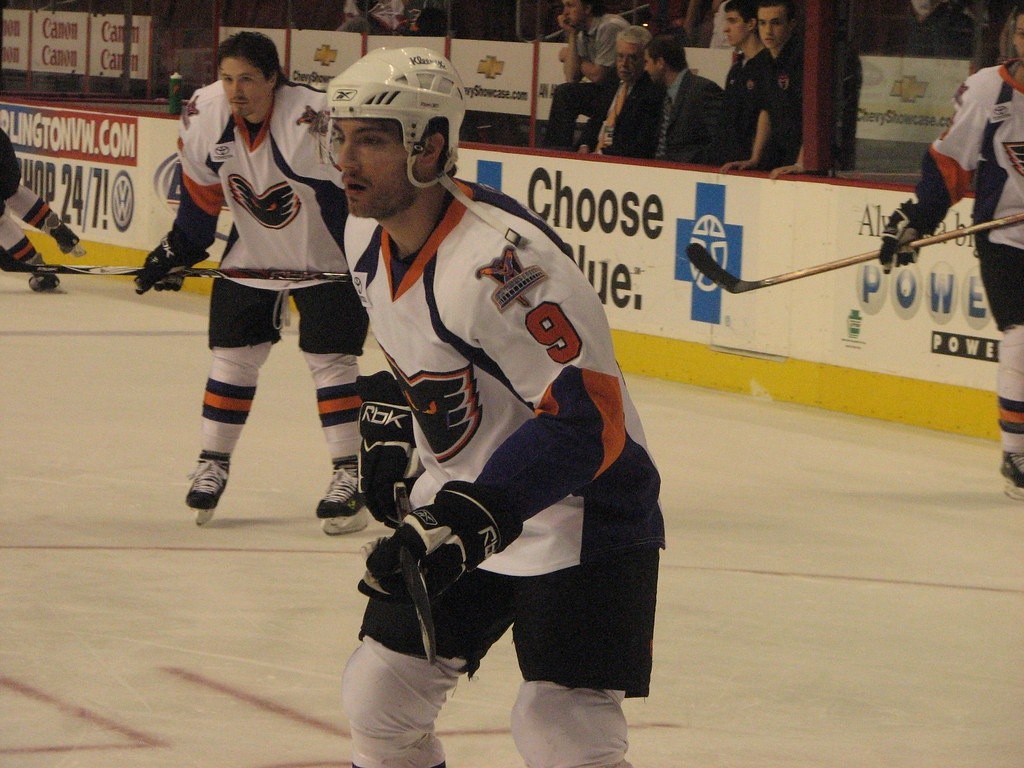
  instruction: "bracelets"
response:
[795,162,803,167]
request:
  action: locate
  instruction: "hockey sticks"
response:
[685,212,1024,294]
[0,246,352,282]
[392,480,437,667]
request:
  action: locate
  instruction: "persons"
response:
[881,0,980,60]
[324,46,666,768]
[542,0,862,179]
[880,0,1024,488]
[133,32,370,518]
[0,126,80,291]
[334,0,446,37]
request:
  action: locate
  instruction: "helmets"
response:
[325,44,463,153]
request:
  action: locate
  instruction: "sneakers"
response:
[316,455,369,537]
[49,224,87,258]
[1000,450,1024,506]
[185,449,231,526]
[25,252,60,293]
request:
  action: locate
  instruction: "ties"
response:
[596,80,633,161]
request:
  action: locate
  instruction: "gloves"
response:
[355,479,523,605]
[134,219,209,294]
[354,372,416,524]
[877,193,948,272]
